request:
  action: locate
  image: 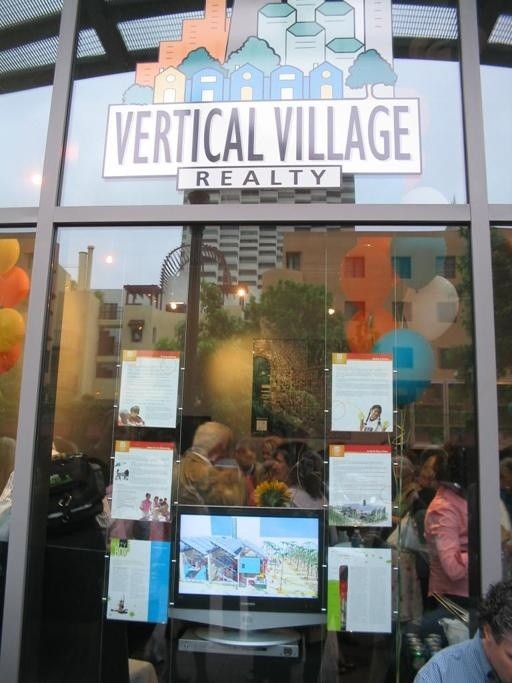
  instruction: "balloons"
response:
[0,238,30,377]
[336,194,460,412]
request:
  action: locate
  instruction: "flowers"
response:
[252,481,298,506]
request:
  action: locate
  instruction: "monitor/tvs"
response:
[167,504,330,647]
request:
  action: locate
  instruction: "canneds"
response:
[404,632,442,680]
